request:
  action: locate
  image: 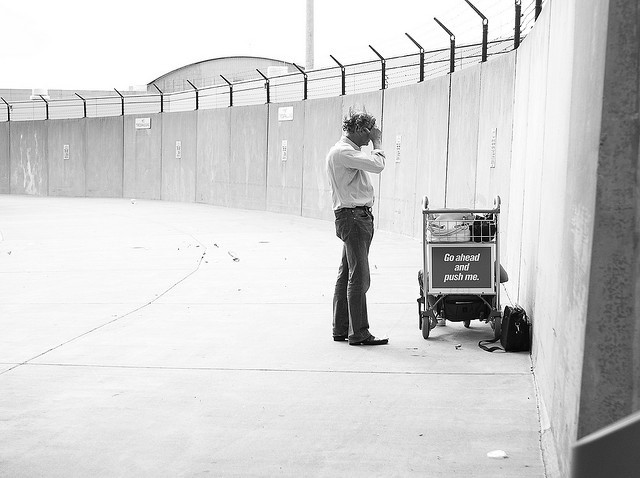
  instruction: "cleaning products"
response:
[417,196,502,338]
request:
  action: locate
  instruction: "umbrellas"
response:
[333,332,350,342]
[349,333,388,346]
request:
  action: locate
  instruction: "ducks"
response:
[436,293,494,322]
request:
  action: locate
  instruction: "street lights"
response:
[476,304,532,353]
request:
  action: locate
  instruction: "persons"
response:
[325,106,389,347]
